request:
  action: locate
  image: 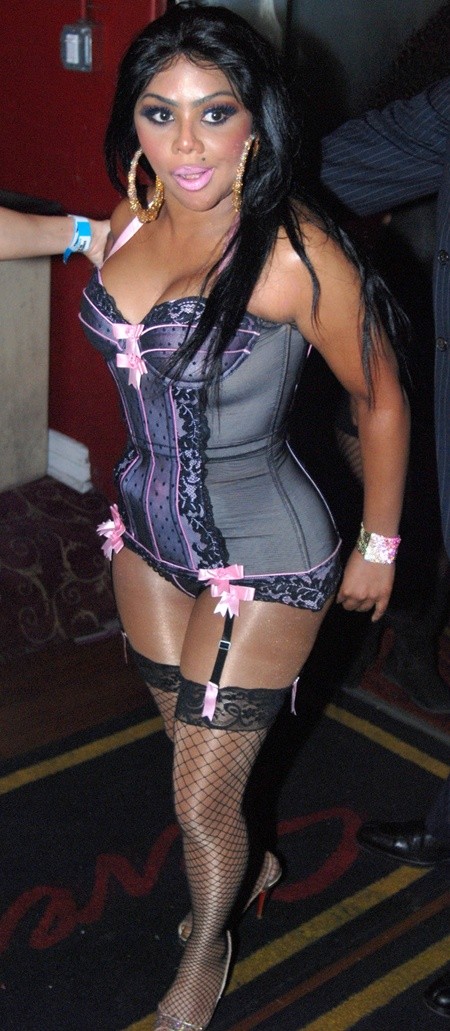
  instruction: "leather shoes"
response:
[354,820,450,869]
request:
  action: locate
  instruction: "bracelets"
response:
[356,521,404,567]
[62,214,92,262]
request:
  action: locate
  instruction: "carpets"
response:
[2,679,448,1031]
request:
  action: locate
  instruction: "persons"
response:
[289,0,449,716]
[0,206,111,270]
[73,5,416,1029]
[279,73,450,867]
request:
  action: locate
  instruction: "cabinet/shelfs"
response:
[1,189,63,493]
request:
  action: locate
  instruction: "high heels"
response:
[153,929,232,1030]
[178,851,283,942]
